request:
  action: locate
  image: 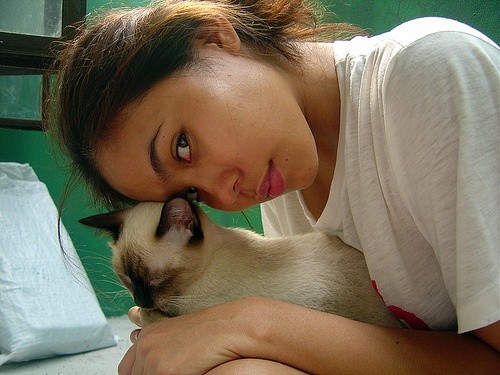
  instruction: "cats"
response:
[78,189,404,330]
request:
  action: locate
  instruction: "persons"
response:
[56,0,500,375]
